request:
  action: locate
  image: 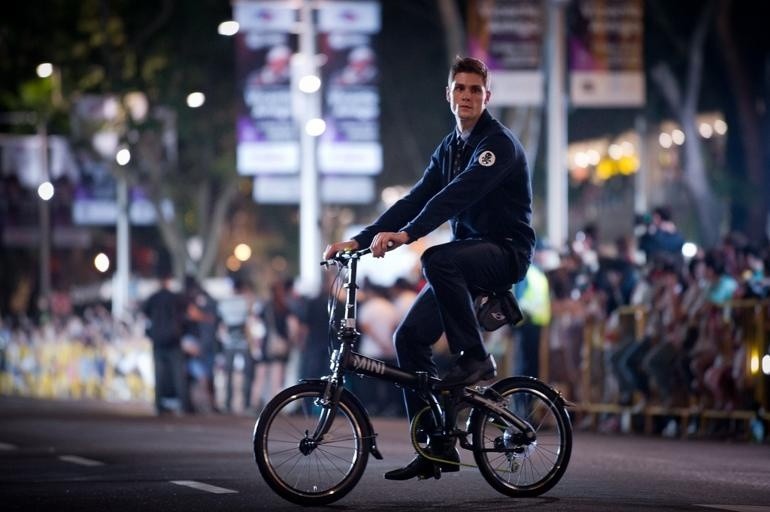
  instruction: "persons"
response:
[320,55,536,481]
[0,202,770,445]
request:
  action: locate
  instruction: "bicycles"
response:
[253,240,577,506]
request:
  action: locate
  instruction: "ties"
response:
[453,135,465,178]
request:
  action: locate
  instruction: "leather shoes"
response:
[431,354,497,392]
[384,446,460,480]
